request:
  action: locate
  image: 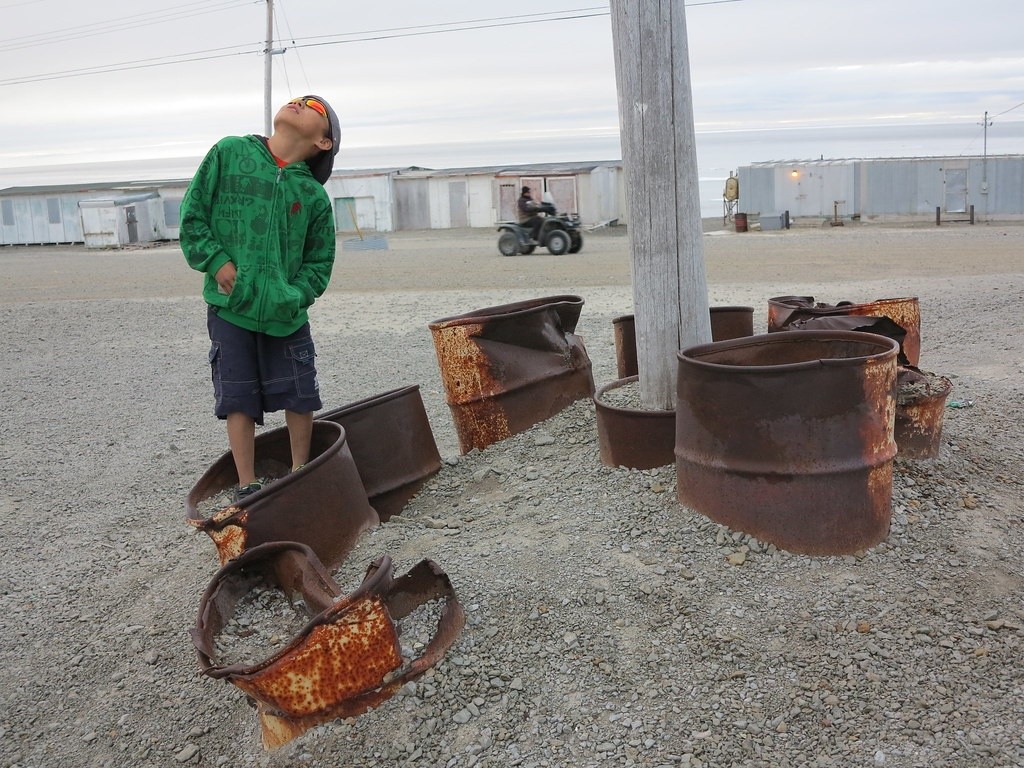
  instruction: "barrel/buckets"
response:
[610,306,755,381]
[308,385,443,523]
[182,418,381,586]
[735,213,748,233]
[428,295,598,456]
[769,294,922,382]
[886,363,949,461]
[671,329,901,559]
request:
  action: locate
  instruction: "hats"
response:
[302,95,341,185]
[522,187,531,193]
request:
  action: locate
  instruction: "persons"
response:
[518,186,556,241]
[180,96,341,488]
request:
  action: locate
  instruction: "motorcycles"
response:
[494,191,586,257]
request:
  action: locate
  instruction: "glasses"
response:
[288,97,332,141]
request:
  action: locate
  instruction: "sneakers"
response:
[288,464,304,476]
[233,483,262,502]
